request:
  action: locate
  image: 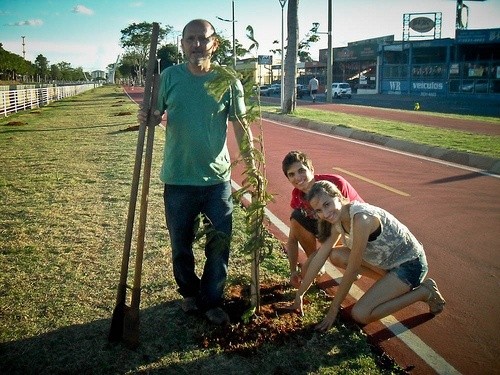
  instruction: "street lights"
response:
[157,57,160,74]
[279,0,287,111]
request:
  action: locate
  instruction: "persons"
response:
[137,19,268,323]
[308,76,319,103]
[279,150,445,335]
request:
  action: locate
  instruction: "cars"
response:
[296,84,310,100]
[272,83,281,94]
[260,85,271,97]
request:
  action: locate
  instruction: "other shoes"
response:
[313,98,316,103]
[423,277,446,314]
[205,307,231,325]
[182,297,197,312]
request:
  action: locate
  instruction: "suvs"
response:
[325,82,351,98]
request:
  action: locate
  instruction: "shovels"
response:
[107,22,162,348]
